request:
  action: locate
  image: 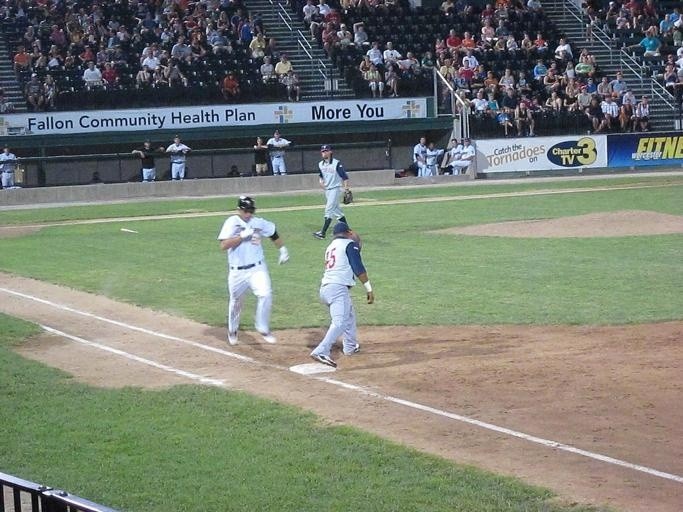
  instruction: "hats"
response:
[333,223,349,233]
[320,145,330,151]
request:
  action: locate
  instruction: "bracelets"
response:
[363,281,373,293]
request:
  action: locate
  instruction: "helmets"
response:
[238,197,255,210]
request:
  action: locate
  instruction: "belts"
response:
[4,171,14,173]
[275,155,282,158]
[232,261,261,269]
[173,161,182,164]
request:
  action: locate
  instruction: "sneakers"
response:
[345,344,359,356]
[259,332,275,343]
[228,331,238,345]
[308,353,337,367]
[313,231,325,239]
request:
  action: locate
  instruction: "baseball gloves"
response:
[344,189,353,204]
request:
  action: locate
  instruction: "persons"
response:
[310,222,375,366]
[218,196,290,346]
[313,143,352,240]
[1,1,683,187]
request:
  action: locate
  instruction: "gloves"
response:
[278,246,290,264]
[240,226,255,240]
